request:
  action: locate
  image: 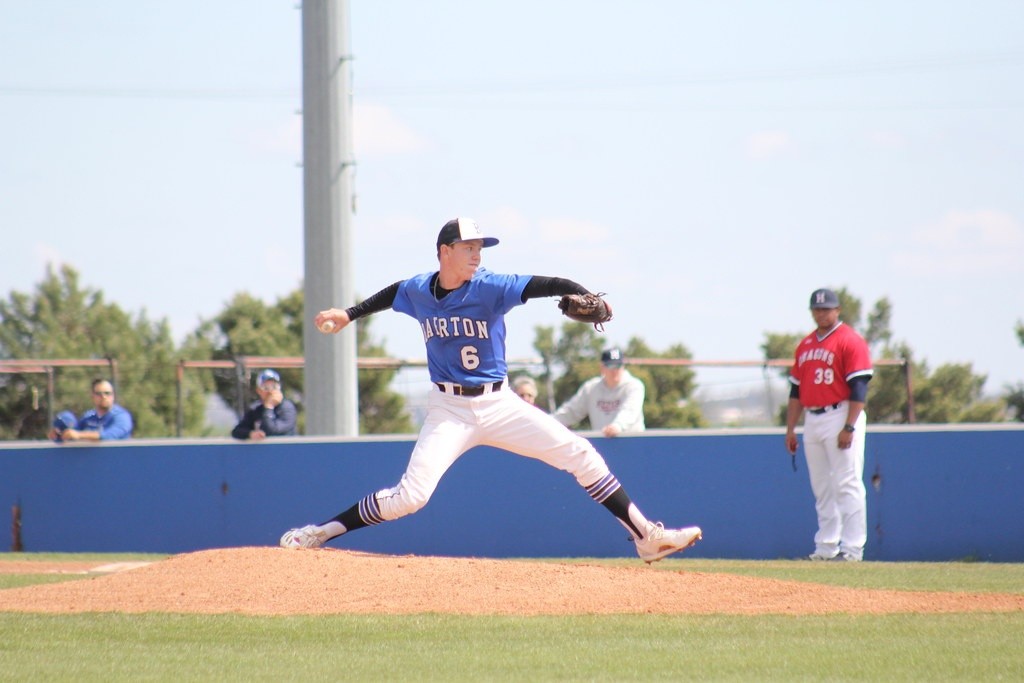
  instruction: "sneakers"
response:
[280,525,327,548]
[634,520,702,565]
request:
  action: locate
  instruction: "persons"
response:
[230,369,297,441]
[786,289,874,561]
[47,378,133,442]
[509,375,540,410]
[549,345,646,437]
[280,217,701,565]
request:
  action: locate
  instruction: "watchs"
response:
[844,424,855,432]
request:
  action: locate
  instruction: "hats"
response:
[437,218,499,261]
[811,289,839,308]
[259,370,279,385]
[601,350,624,364]
[512,377,538,396]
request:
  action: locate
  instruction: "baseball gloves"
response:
[557,293,614,325]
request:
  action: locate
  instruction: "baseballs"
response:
[318,320,334,334]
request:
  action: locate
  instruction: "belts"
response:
[435,381,502,395]
[810,403,838,414]
